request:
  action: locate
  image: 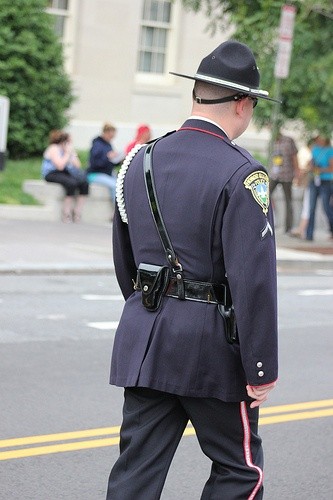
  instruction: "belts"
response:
[135,269,232,305]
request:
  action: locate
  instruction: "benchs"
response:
[22,180,113,225]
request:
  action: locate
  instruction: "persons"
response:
[41,124,150,224]
[106,39,282,500]
[265,118,333,242]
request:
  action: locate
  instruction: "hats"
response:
[168,41,282,105]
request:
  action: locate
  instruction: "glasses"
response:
[234,94,258,108]
